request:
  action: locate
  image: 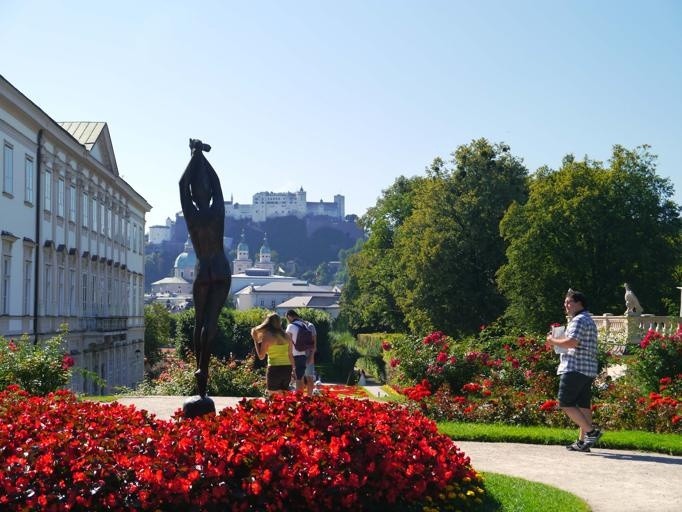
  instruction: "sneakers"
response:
[565,429,601,452]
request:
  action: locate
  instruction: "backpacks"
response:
[288,321,312,352]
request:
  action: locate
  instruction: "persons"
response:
[250,313,296,390]
[179,138,234,400]
[284,309,313,397]
[302,318,317,396]
[544,287,603,453]
